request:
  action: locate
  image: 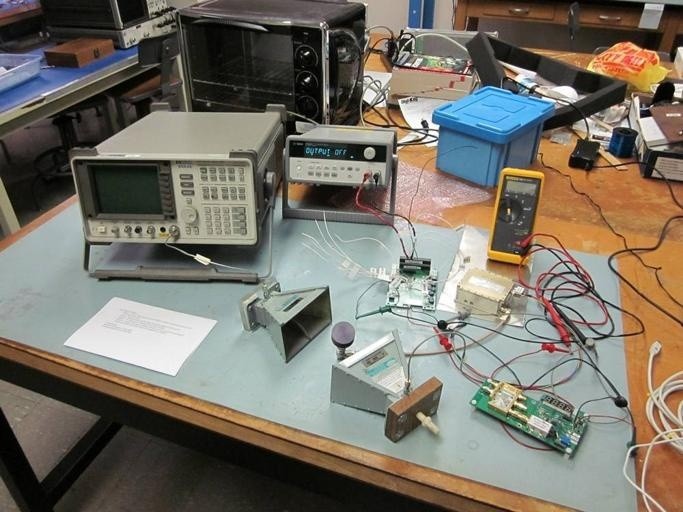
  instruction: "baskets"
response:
[1,54,42,92]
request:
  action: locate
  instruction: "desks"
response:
[454,0,683,54]
[0,40,145,240]
[1,33,683,512]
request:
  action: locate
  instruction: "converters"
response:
[569,138,601,171]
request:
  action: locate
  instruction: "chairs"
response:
[111,33,183,121]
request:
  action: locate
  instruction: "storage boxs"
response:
[431,85,556,189]
